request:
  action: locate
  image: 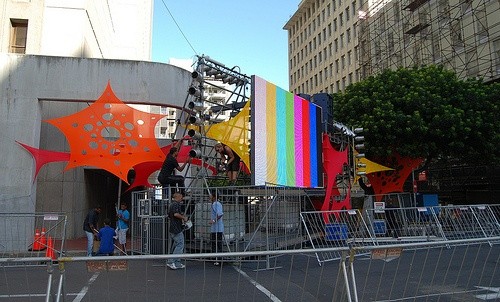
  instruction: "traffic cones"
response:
[40,237,58,264]
[28,228,47,251]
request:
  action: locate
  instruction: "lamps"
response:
[334,123,366,177]
[181,57,248,158]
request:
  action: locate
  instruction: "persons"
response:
[83,206,103,256]
[95,219,115,256]
[215,143,240,185]
[158,147,189,198]
[116,202,129,254]
[167,192,188,269]
[210,192,224,265]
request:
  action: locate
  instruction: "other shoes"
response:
[215,262,220,265]
[176,263,185,269]
[167,262,176,269]
